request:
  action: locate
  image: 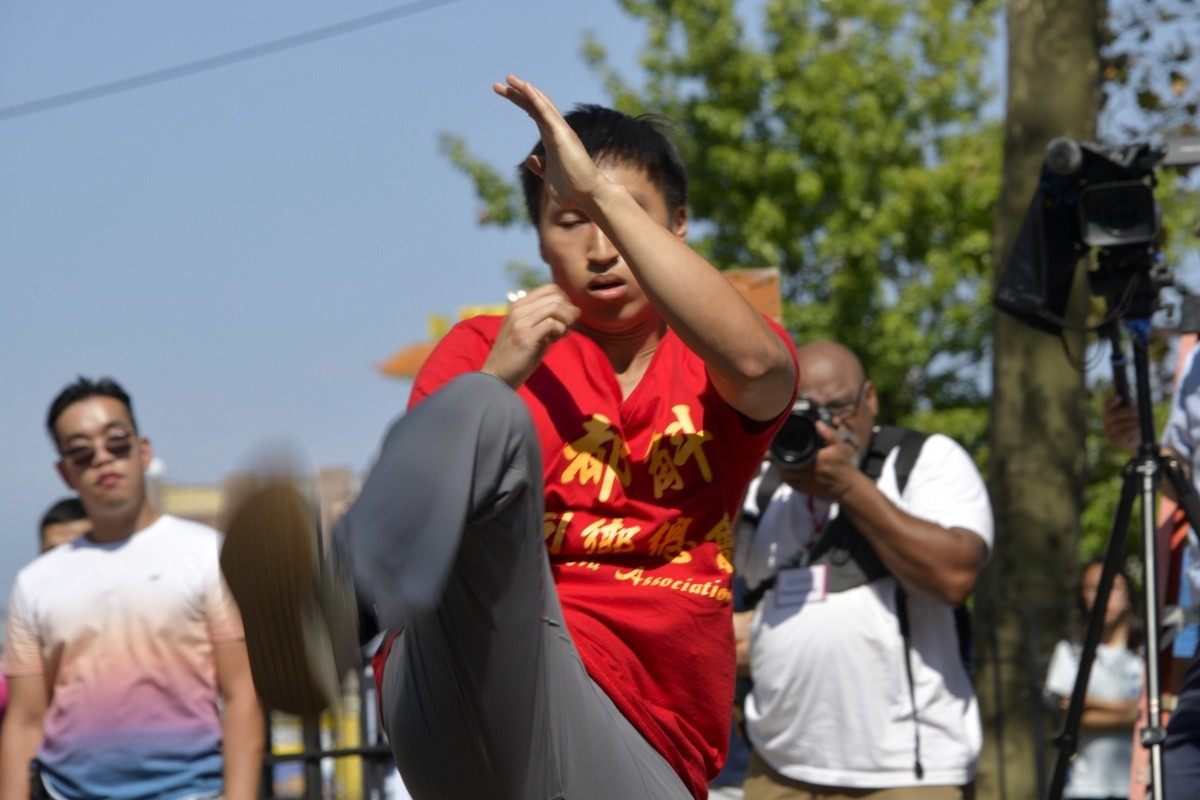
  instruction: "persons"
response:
[0,376,266,800]
[731,339,995,800]
[217,72,801,800]
[1102,342,1200,800]
[1045,562,1146,800]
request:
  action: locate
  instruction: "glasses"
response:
[67,430,138,468]
[832,380,868,421]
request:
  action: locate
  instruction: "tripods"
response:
[1045,286,1200,799]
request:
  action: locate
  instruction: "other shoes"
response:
[215,475,362,716]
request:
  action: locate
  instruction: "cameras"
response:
[769,395,835,472]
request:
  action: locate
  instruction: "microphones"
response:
[1047,138,1087,178]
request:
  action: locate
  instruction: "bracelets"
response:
[829,482,853,501]
